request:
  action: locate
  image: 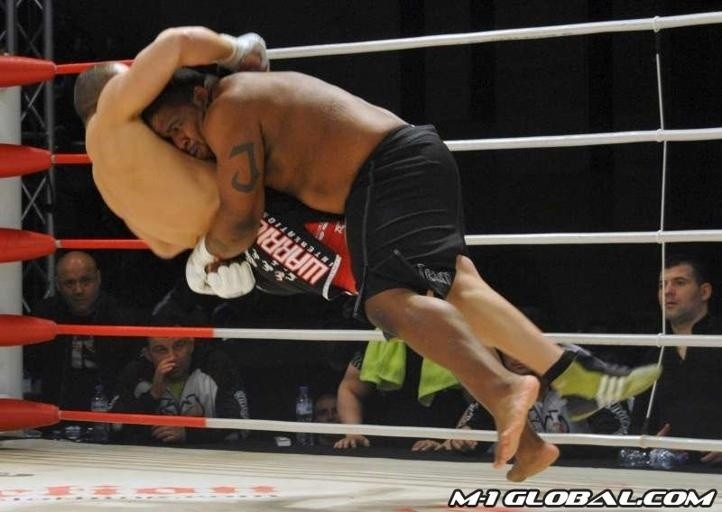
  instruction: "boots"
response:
[549,351,662,424]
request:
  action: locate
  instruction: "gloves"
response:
[219,32,270,71]
[186,234,257,299]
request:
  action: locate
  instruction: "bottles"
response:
[296,386,314,448]
[91,385,110,442]
